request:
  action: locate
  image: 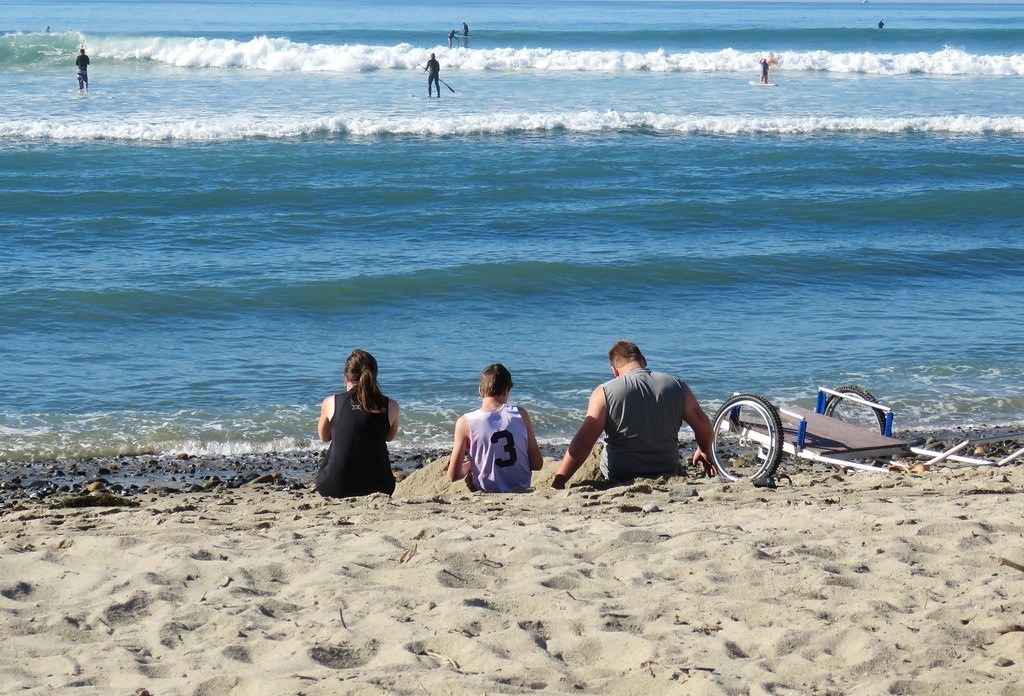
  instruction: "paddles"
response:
[418,63,457,93]
[457,30,460,50]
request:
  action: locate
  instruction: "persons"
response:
[878,19,885,30]
[46,25,52,34]
[759,58,769,85]
[448,364,543,493]
[317,349,400,500]
[425,54,440,98]
[550,340,719,490]
[448,22,469,49]
[76,49,90,93]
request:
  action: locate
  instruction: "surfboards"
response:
[749,80,779,88]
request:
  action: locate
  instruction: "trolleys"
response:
[708,386,1024,484]
